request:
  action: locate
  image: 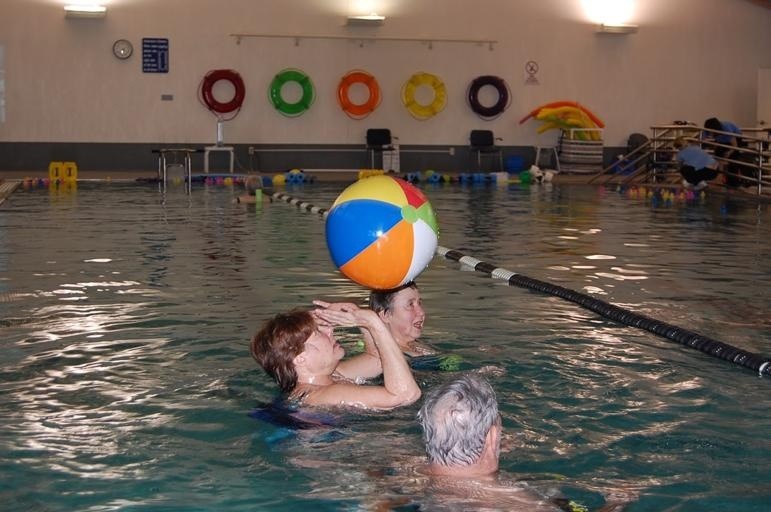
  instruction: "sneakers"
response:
[682,179,690,188]
[694,180,708,191]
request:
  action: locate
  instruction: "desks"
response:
[534,145,561,173]
[203,146,235,175]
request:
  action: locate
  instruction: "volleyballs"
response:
[323,175,440,290]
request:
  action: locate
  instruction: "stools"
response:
[49,161,78,183]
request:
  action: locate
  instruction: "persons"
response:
[368,280,440,360]
[609,117,770,195]
[403,369,567,512]
[249,299,421,412]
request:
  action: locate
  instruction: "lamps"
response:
[597,23,639,36]
[345,15,386,27]
[63,4,108,20]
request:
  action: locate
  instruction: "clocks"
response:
[113,39,133,59]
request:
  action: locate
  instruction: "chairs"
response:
[364,127,396,169]
[469,129,504,173]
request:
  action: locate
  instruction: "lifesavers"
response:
[468,75,508,117]
[338,72,379,115]
[270,71,313,114]
[202,69,245,113]
[404,73,448,118]
